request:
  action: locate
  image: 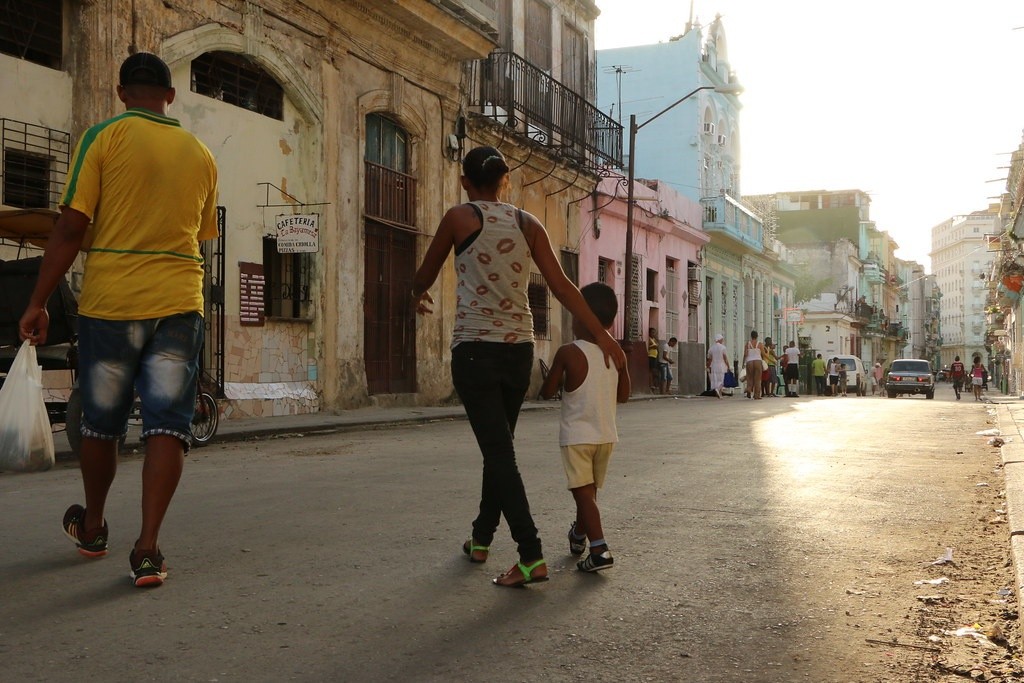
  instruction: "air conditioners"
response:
[718,134,729,147]
[688,267,700,280]
[704,123,717,135]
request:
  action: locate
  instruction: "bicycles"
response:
[956,381,961,400]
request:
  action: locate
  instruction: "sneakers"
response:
[568,523,587,557]
[577,551,614,572]
[63,504,110,557]
[128,543,168,586]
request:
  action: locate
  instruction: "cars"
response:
[886,359,935,399]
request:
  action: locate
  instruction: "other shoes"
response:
[747,389,751,398]
[755,397,763,399]
[784,392,799,397]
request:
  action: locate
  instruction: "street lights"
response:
[621,83,745,397]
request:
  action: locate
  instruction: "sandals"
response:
[463,537,491,562]
[492,560,551,586]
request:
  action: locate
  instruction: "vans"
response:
[824,355,868,397]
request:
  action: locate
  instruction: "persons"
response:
[812,354,848,397]
[706,334,730,399]
[659,337,677,395]
[948,356,965,399]
[742,331,804,400]
[969,356,988,401]
[539,282,630,573]
[408,145,628,588]
[870,363,882,396]
[19,52,221,587]
[648,327,659,390]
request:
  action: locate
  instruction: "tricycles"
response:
[0,205,220,455]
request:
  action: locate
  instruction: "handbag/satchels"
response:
[0,337,57,472]
[724,371,738,387]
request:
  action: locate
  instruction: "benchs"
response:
[1,257,78,370]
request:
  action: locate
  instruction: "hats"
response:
[715,334,723,341]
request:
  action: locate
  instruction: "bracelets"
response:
[411,290,426,299]
